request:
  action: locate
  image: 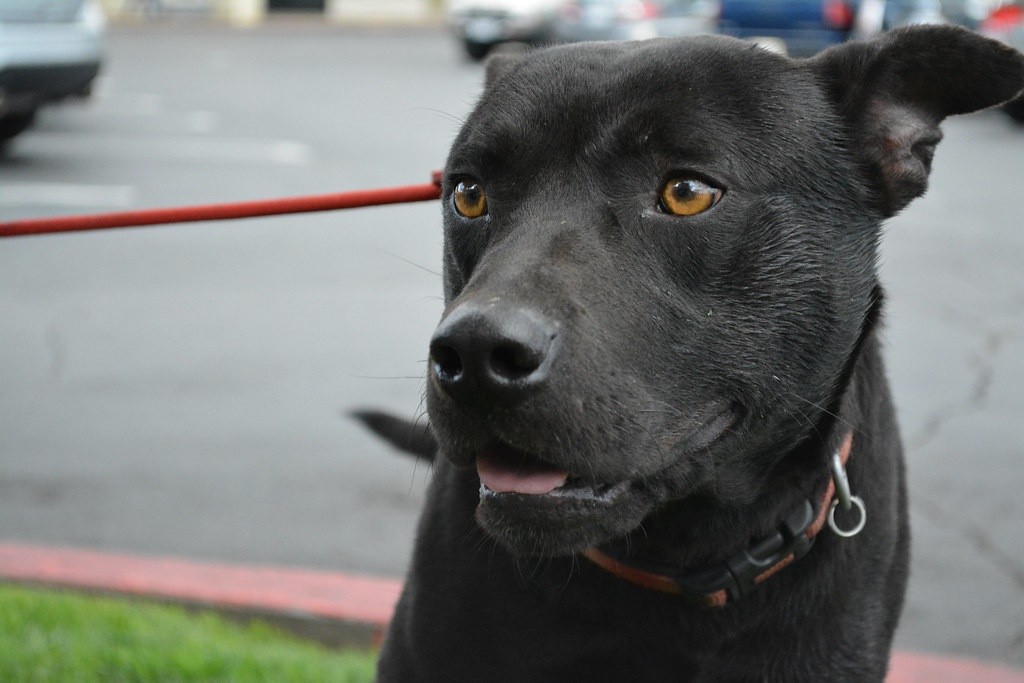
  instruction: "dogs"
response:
[344,22,1024,683]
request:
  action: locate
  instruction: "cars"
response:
[447,0,1024,74]
